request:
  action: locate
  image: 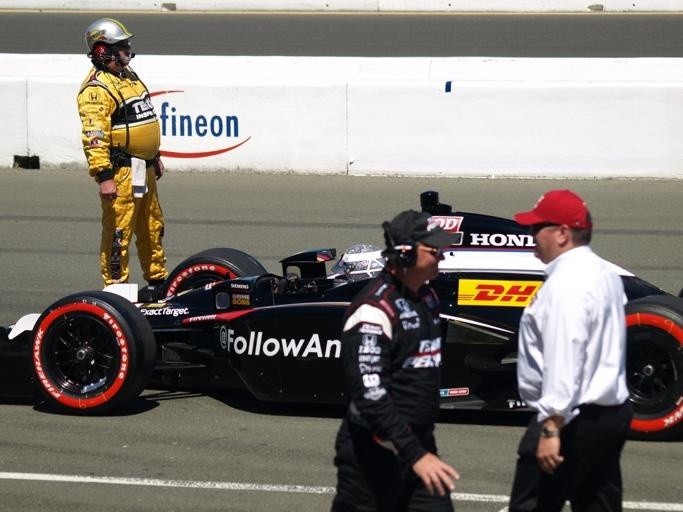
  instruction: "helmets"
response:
[85,19,135,70]
[331,245,383,282]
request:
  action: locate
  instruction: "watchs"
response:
[540,427,561,437]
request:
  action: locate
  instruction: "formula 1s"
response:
[0,191,683,439]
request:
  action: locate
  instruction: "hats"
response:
[383,213,452,273]
[515,190,592,229]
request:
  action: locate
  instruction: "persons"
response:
[508,190,633,512]
[77,18,168,303]
[330,210,461,512]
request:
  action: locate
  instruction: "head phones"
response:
[382,220,418,270]
[87,43,112,64]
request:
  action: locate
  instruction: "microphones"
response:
[105,52,135,62]
